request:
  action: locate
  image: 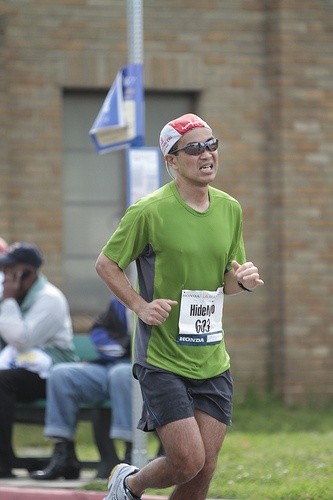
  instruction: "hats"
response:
[0,242,43,268]
[159,113,212,180]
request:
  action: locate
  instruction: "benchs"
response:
[0,329,152,484]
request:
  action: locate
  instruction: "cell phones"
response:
[21,270,31,280]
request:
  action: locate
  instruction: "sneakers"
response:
[103,463,142,500]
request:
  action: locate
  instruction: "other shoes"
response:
[31,441,80,480]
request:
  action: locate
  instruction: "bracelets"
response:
[236,280,252,292]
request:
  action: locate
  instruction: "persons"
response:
[93,111,264,500]
[1,240,77,482]
[28,291,135,483]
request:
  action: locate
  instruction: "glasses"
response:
[170,138,218,156]
